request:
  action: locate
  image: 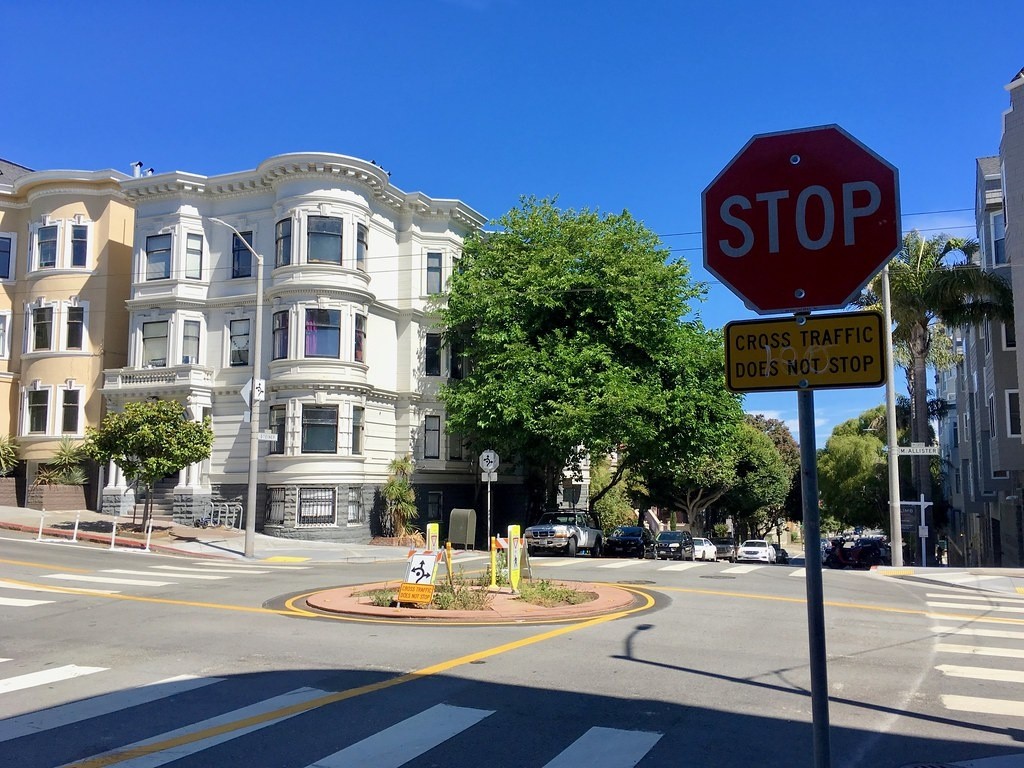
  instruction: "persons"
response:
[937,545,944,564]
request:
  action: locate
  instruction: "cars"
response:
[856,537,883,556]
[601,527,656,559]
[771,544,790,564]
[691,538,718,561]
[737,539,776,564]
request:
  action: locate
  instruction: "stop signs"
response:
[702,124,904,313]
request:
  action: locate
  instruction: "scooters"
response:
[826,537,868,571]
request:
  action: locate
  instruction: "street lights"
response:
[207,215,264,561]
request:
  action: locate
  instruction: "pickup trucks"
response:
[524,511,605,559]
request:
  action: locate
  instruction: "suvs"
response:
[712,536,737,563]
[652,530,694,560]
